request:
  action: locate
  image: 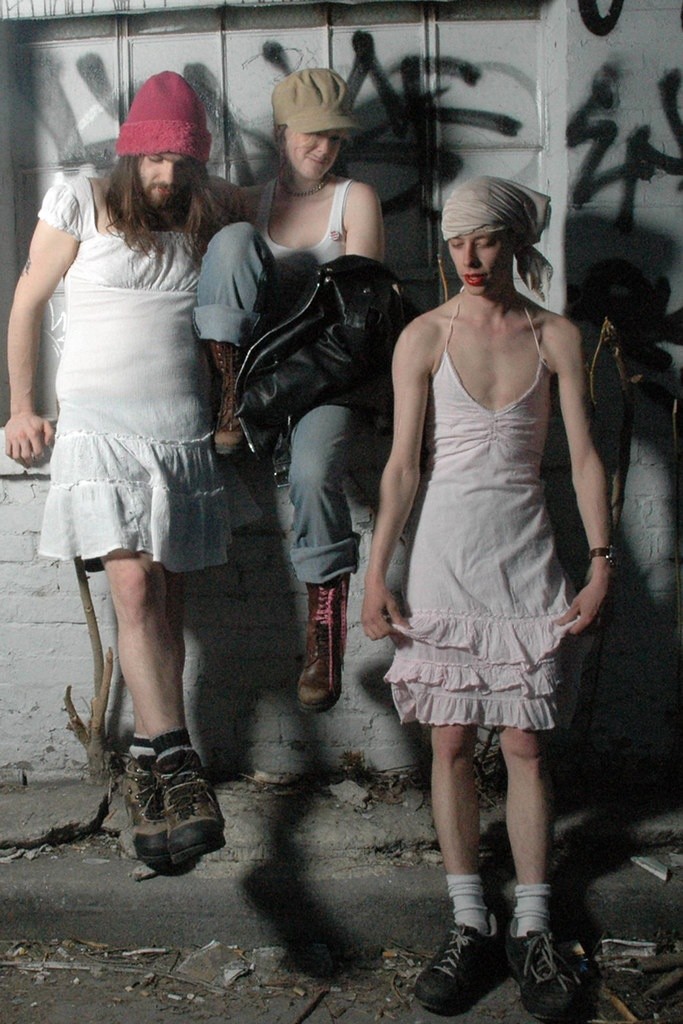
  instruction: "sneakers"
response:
[415,914,504,1010]
[505,918,584,1021]
[124,748,225,864]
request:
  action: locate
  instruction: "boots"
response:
[298,574,350,714]
[211,341,248,454]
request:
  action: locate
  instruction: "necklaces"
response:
[278,171,331,197]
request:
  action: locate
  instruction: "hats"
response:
[117,72,212,163]
[272,69,362,133]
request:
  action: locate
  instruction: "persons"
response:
[363,174,618,1022]
[1,69,249,868]
[191,66,387,716]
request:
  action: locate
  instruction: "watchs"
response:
[588,545,617,568]
